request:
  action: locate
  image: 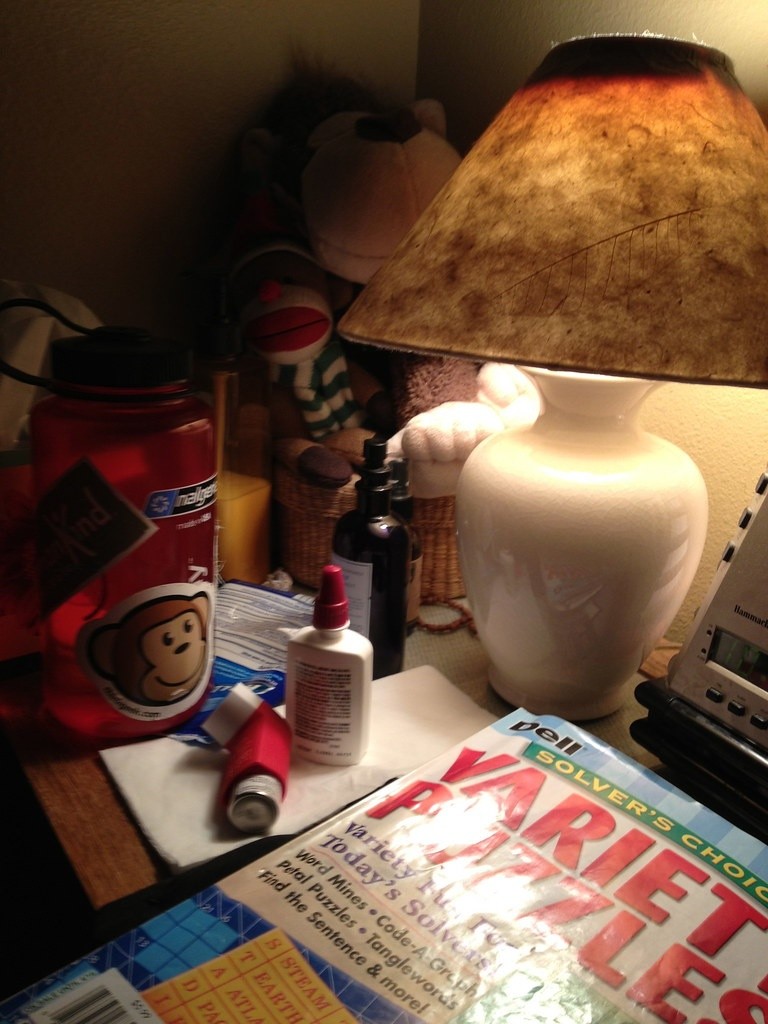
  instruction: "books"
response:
[2,706,767,1023]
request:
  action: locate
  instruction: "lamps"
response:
[337,36,767,719]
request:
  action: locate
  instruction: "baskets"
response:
[269,459,467,604]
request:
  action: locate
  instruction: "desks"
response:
[9,592,667,910]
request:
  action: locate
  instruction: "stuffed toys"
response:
[217,70,463,491]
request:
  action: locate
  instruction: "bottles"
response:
[332,434,424,680]
[0,297,221,743]
